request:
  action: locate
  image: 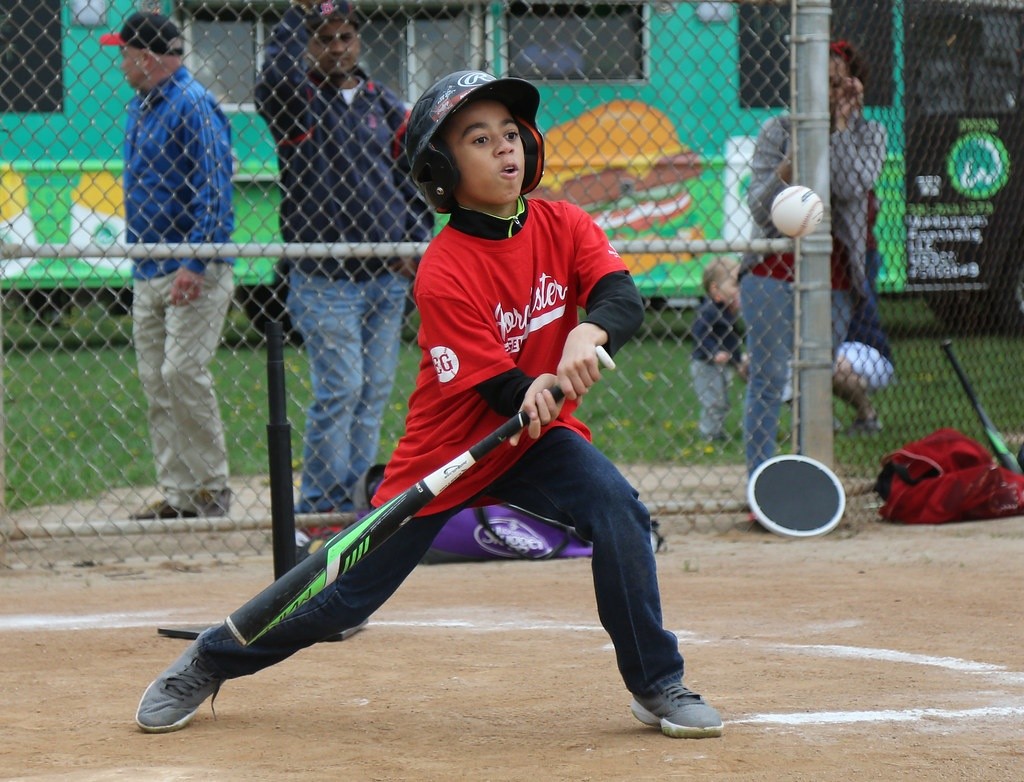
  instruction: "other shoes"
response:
[129,486,233,519]
[700,430,731,444]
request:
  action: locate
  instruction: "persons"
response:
[254,1,433,510]
[736,41,886,476]
[102,10,233,515]
[781,276,895,431]
[692,257,747,442]
[137,69,726,738]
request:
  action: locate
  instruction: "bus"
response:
[0,0,1023,335]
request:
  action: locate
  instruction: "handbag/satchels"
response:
[873,428,1023,524]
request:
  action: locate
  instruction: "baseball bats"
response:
[224,344,616,648]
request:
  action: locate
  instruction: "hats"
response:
[303,0,358,35]
[100,11,182,55]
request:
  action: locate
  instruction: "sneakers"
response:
[631,678,724,739]
[833,408,882,437]
[295,510,344,547]
[138,628,226,735]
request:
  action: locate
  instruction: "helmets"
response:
[405,69,545,214]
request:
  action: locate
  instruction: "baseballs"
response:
[769,184,825,239]
